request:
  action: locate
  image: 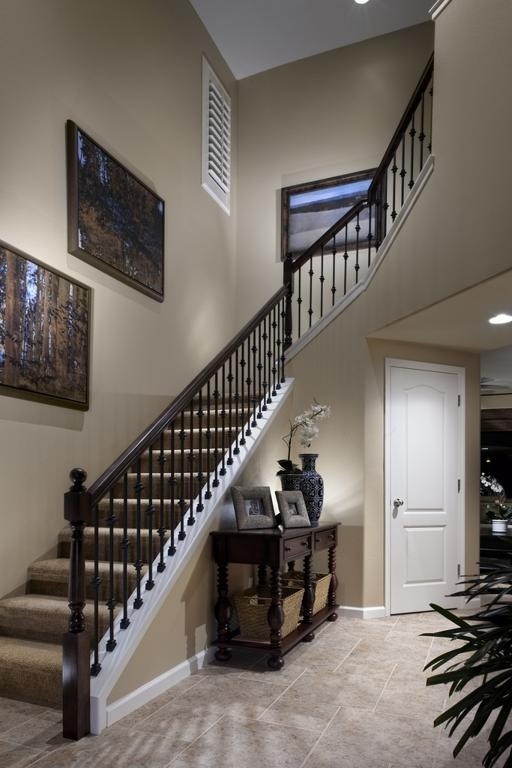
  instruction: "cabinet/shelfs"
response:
[210,519,343,669]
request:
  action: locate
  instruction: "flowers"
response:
[277,401,331,469]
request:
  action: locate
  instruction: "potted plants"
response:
[483,495,512,535]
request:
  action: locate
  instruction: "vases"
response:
[299,452,325,523]
[282,473,306,496]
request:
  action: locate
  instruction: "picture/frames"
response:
[231,486,277,531]
[67,119,165,305]
[0,240,91,412]
[276,491,311,529]
[281,167,386,263]
